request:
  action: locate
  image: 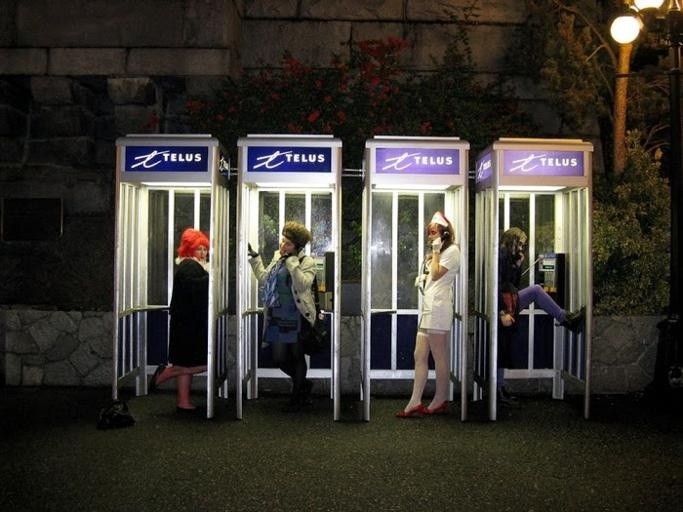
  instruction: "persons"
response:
[395,211,461,418]
[497,226,586,409]
[247,220,317,412]
[148,227,209,417]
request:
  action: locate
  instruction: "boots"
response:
[562,305,587,334]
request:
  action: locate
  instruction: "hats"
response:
[430,211,448,227]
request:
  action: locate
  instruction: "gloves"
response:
[284,249,297,258]
[415,274,428,288]
[432,237,444,253]
[248,243,258,257]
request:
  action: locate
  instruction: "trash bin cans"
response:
[651,318,683,398]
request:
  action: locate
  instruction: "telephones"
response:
[430,231,449,250]
[288,246,303,256]
[513,250,523,260]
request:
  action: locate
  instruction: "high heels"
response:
[395,403,424,417]
[424,400,448,414]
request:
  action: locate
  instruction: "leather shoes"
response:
[280,378,313,412]
[150,364,166,392]
[176,404,206,414]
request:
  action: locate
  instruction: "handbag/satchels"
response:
[501,291,518,317]
[301,314,323,344]
[97,400,134,429]
